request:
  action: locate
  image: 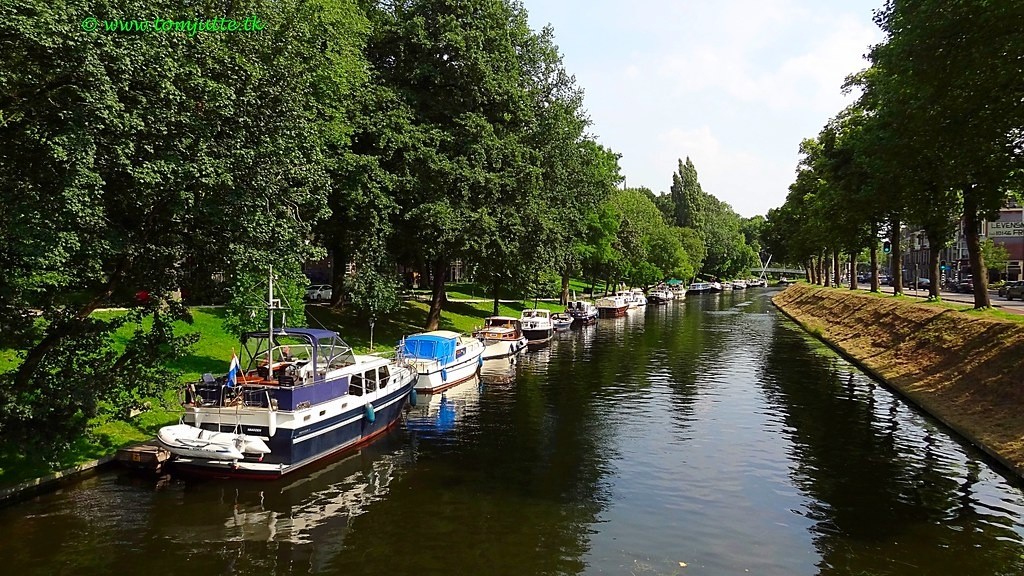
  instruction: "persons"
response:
[277,346,293,361]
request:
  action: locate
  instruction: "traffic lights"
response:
[883,241,891,252]
[940,260,946,270]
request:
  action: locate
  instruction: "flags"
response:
[226,353,242,387]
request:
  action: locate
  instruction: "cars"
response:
[998,281,1017,297]
[1006,281,1024,301]
[857,272,890,284]
[963,275,973,279]
[908,278,932,291]
[303,284,333,300]
[955,279,974,293]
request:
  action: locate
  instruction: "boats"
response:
[551,313,574,332]
[157,263,419,483]
[671,284,687,302]
[721,281,733,292]
[519,309,556,344]
[632,288,646,306]
[748,278,766,288]
[615,283,638,309]
[647,282,674,303]
[777,277,787,286]
[471,316,528,358]
[687,282,723,295]
[394,331,486,392]
[566,300,599,325]
[596,297,630,318]
[734,280,747,290]
